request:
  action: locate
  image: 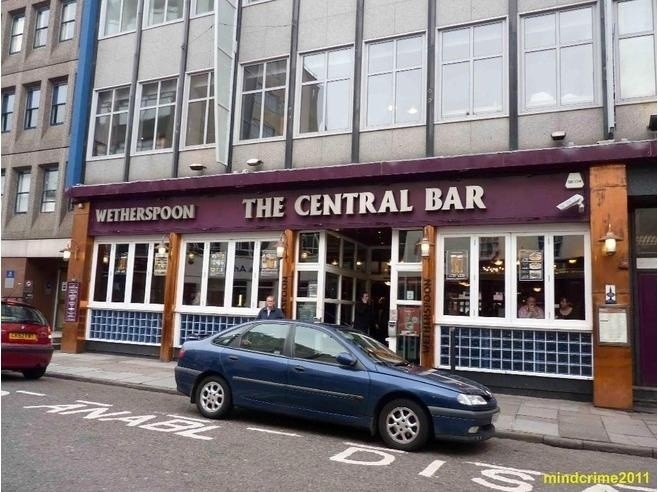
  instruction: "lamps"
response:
[273,233,288,260]
[103,249,109,264]
[59,239,79,262]
[596,214,623,256]
[551,131,566,140]
[186,246,199,264]
[154,233,168,260]
[246,159,263,166]
[646,115,656,133]
[188,164,206,170]
[416,227,435,259]
[78,203,84,209]
[300,249,311,259]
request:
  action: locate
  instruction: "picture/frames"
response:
[596,304,632,347]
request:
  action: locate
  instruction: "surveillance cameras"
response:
[556,194,585,212]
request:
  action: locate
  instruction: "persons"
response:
[517,296,544,320]
[10,297,27,319]
[554,296,583,320]
[256,295,285,321]
[354,291,388,345]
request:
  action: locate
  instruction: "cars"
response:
[1,291,56,380]
[172,319,503,453]
